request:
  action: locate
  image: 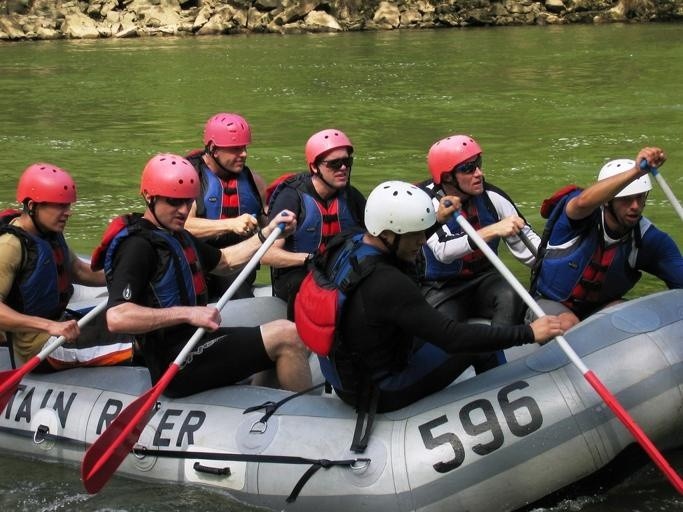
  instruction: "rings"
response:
[246,226,250,232]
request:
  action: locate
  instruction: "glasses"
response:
[454,157,482,174]
[319,156,354,169]
[164,198,194,206]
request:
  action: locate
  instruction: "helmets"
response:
[138,153,201,199]
[598,159,653,200]
[427,135,482,184]
[203,113,253,149]
[364,180,436,237]
[16,162,77,204]
[304,129,354,173]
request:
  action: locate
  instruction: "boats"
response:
[0,252,683,512]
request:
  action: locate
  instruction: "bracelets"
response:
[258,230,265,243]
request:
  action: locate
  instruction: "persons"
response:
[295,180,564,413]
[523,147,683,331]
[90,154,313,399]
[184,113,268,303]
[261,128,366,323]
[416,135,541,326]
[0,163,145,374]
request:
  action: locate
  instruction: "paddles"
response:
[0,292,109,416]
[81,211,290,496]
[443,200,683,494]
